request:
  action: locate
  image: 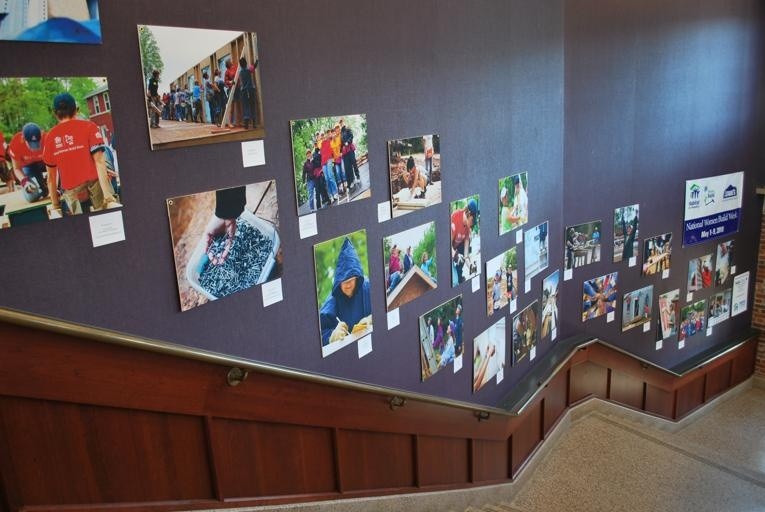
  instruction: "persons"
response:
[500,175,528,233]
[473,344,495,392]
[451,199,478,289]
[146,55,260,129]
[319,236,372,346]
[566,215,729,337]
[0,92,119,215]
[407,135,434,199]
[203,186,246,266]
[427,304,463,369]
[492,264,515,313]
[386,244,437,296]
[302,117,360,213]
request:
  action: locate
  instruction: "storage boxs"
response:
[186,209,280,302]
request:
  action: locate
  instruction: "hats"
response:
[306,117,343,155]
[54,92,75,112]
[24,123,41,150]
[467,199,477,219]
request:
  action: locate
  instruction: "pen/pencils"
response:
[336,317,350,335]
